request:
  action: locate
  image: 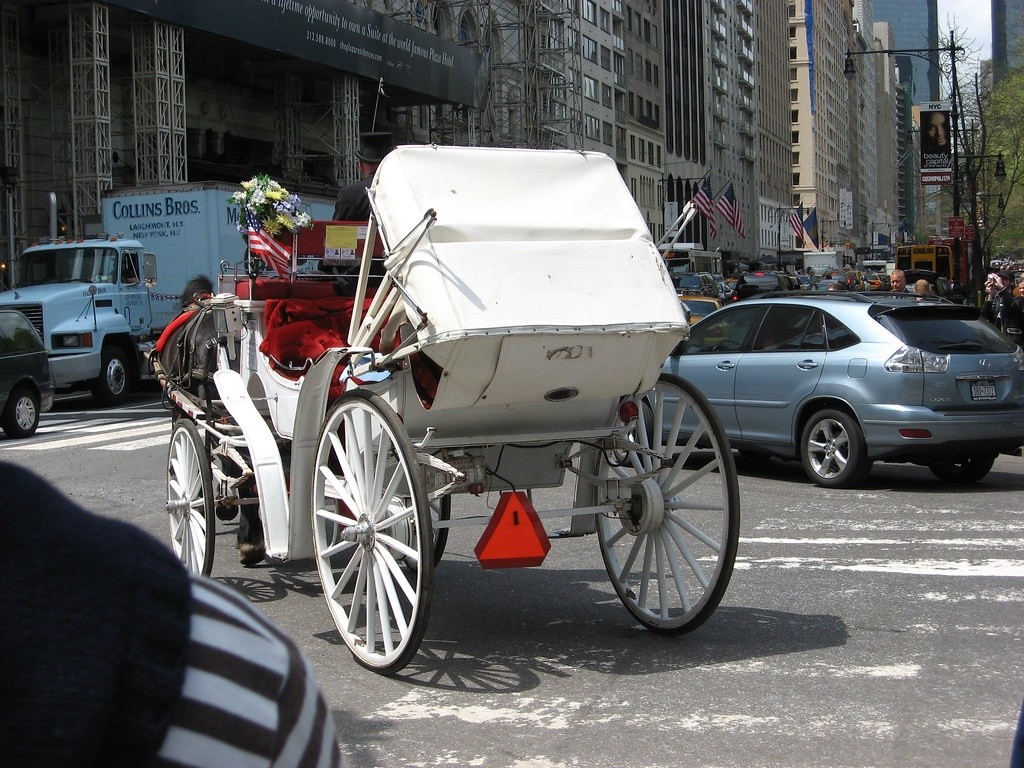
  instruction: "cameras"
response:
[988,275,994,285]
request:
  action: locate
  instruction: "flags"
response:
[691,174,716,240]
[246,209,292,279]
[803,207,819,249]
[715,181,745,238]
[790,201,804,243]
[896,220,912,240]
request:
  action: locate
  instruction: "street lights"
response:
[845,30,1007,299]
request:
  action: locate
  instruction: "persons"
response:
[891,270,911,299]
[982,263,1024,352]
[752,263,775,271]
[318,133,393,288]
[949,278,958,290]
[0,459,345,768]
[921,111,951,169]
[110,256,137,283]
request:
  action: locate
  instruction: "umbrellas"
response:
[744,255,780,266]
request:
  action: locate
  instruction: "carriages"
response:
[136,142,741,674]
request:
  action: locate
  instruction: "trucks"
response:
[0,180,334,413]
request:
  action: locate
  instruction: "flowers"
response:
[228,173,314,239]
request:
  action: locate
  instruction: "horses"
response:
[158,275,271,569]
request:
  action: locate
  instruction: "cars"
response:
[678,294,733,348]
[602,289,1023,492]
[668,262,950,306]
[0,310,54,438]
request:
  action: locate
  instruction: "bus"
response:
[894,243,956,281]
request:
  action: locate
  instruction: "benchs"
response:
[258,296,395,392]
